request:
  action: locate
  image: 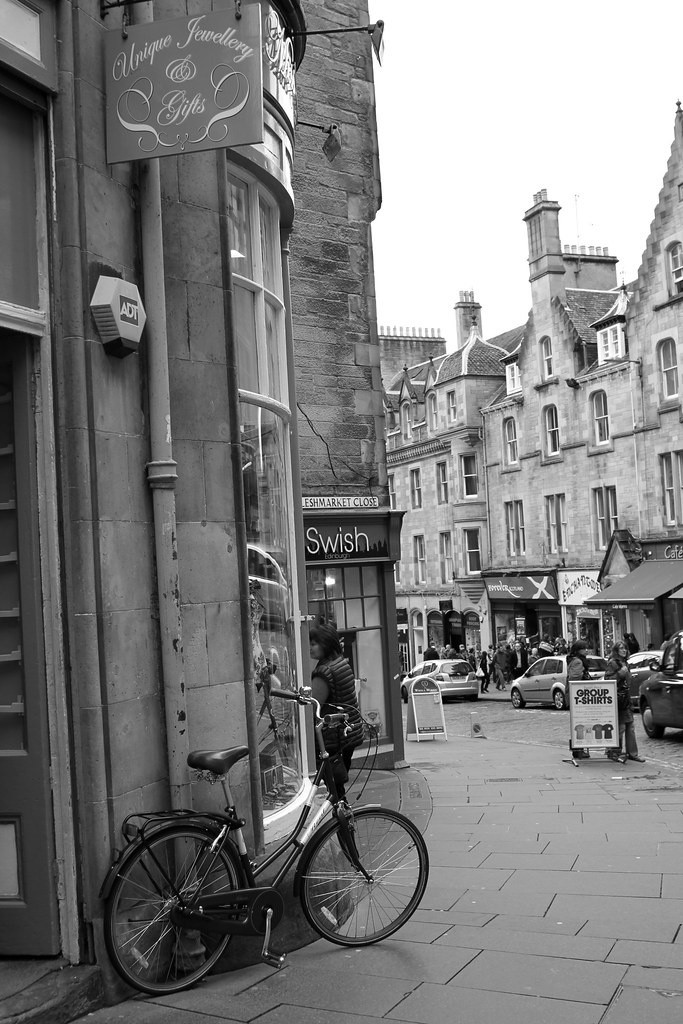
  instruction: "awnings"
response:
[584,559,683,608]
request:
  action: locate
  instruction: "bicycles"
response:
[255,663,298,770]
[97,687,430,993]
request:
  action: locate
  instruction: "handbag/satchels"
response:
[476,666,485,677]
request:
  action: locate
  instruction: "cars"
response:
[624,649,678,712]
[247,544,290,630]
[510,655,610,711]
[636,630,683,740]
[400,657,479,703]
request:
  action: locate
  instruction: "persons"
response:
[604,640,645,763]
[659,632,671,649]
[424,633,567,693]
[310,625,365,804]
[565,641,594,758]
[623,633,640,655]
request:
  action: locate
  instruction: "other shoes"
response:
[496,687,502,690]
[573,751,590,759]
[484,688,489,692]
[628,755,646,762]
[502,687,506,690]
[608,752,623,759]
[481,692,486,694]
